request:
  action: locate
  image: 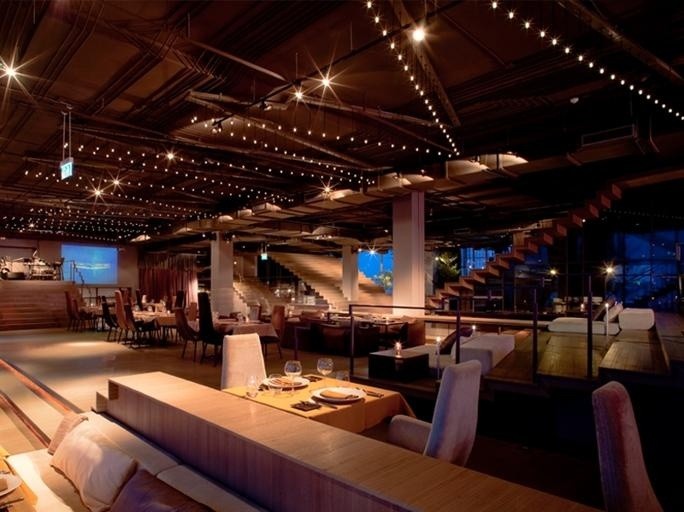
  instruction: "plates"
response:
[309,387,366,404]
[262,375,310,390]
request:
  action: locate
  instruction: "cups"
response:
[131,305,168,316]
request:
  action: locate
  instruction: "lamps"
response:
[210,96,272,135]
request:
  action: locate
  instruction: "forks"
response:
[353,385,384,400]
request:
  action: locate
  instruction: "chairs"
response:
[175,291,285,367]
[220,333,267,390]
[375,360,481,466]
[591,380,664,512]
[547,295,654,335]
[269,311,514,384]
[64,287,197,349]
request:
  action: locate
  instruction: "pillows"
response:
[47,411,88,456]
[104,469,216,512]
[48,419,138,512]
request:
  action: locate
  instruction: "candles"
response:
[606,303,608,336]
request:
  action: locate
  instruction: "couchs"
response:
[6,409,268,512]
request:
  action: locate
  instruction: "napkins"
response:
[291,400,322,412]
[303,375,323,382]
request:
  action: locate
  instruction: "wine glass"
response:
[245,376,259,400]
[268,358,351,400]
